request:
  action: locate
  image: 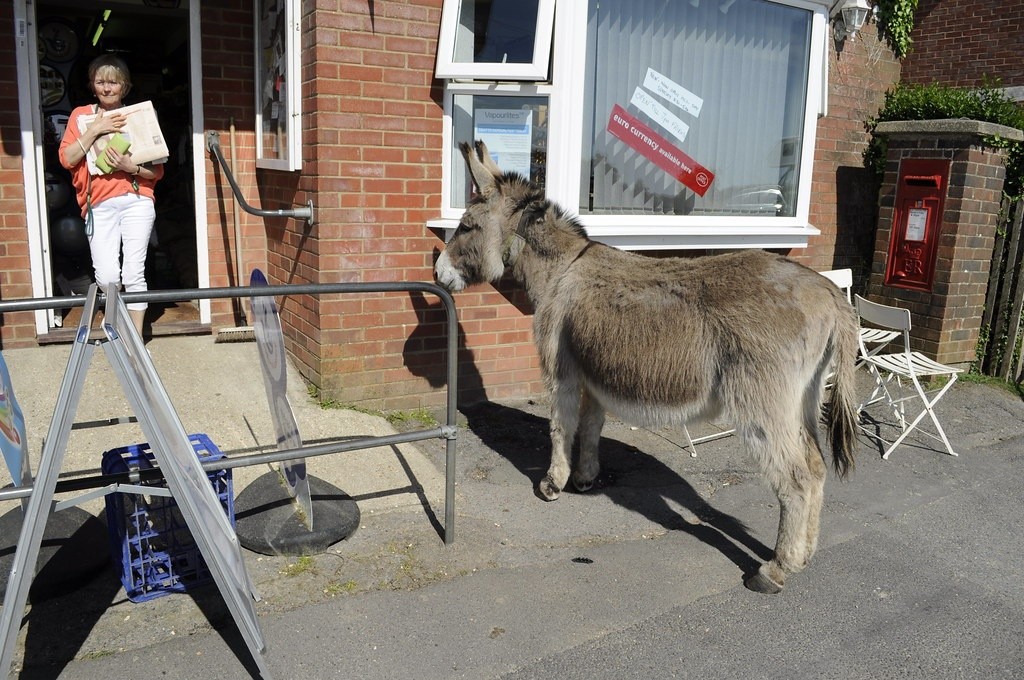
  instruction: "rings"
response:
[109,156,115,163]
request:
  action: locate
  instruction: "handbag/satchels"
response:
[95,132,131,174]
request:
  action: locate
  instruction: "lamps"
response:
[833,1,873,43]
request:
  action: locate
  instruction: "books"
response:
[77,100,169,176]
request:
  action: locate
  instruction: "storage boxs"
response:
[100,433,235,604]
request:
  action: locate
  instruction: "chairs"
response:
[852,293,965,459]
[819,268,902,390]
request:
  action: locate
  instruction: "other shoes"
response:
[145,346,152,360]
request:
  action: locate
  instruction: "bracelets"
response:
[130,165,140,191]
[77,138,87,155]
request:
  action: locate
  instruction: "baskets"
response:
[100,432,236,603]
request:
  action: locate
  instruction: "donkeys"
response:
[434,140,861,596]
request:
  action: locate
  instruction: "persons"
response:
[59,54,164,360]
